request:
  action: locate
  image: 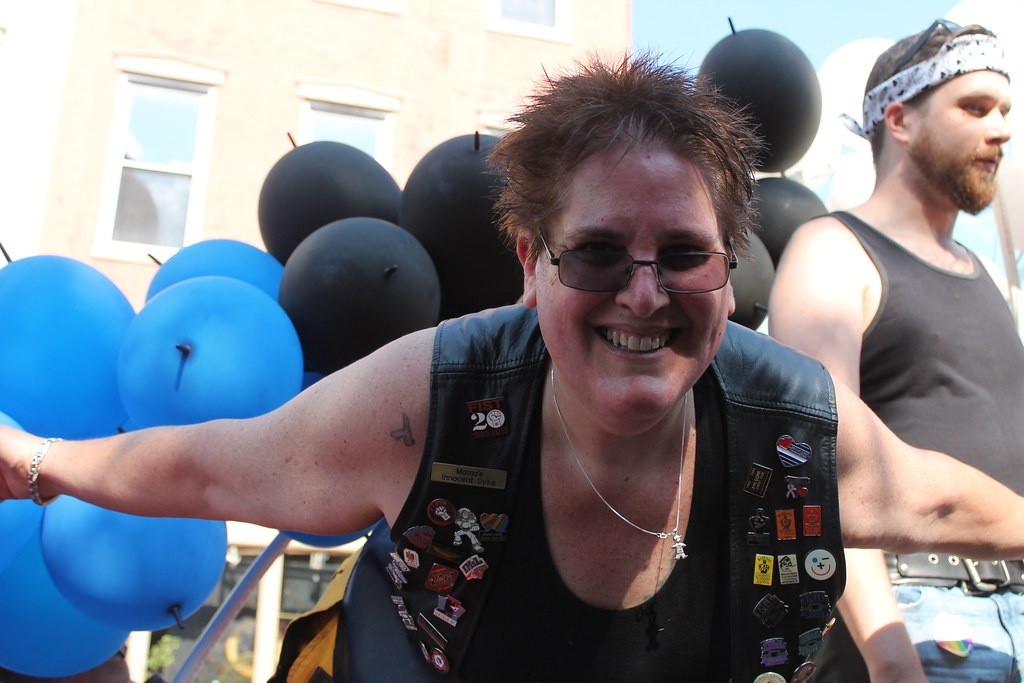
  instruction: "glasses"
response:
[537,228,738,293]
[891,19,961,76]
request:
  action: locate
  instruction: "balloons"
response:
[280,517,384,548]
[694,27,828,329]
[258,134,525,373]
[0,240,302,440]
[0,496,227,679]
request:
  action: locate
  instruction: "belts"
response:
[884,552,1024,595]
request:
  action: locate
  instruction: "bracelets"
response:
[28,438,62,505]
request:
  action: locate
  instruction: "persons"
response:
[770,19,1024,683]
[0,53,1024,683]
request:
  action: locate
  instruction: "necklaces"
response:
[548,364,688,562]
